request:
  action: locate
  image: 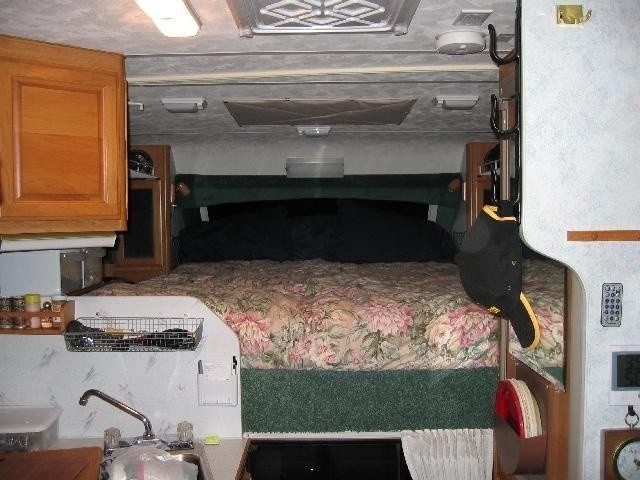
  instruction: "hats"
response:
[454,200,541,351]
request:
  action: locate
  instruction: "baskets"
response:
[63,317,202,351]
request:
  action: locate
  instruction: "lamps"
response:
[431,95,480,111]
[170,182,191,203]
[134,0,202,38]
[297,126,331,137]
[285,158,345,180]
[160,98,207,114]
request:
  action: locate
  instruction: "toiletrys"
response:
[0,295,67,330]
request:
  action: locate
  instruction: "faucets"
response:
[78,390,153,436]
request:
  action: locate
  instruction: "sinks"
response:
[100,441,215,480]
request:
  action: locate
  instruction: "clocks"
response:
[601,428,640,480]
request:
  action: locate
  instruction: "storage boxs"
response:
[0,407,62,452]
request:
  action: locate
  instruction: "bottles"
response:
[25,294,67,329]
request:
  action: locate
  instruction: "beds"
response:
[76,259,502,370]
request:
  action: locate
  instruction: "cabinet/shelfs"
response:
[102,143,170,282]
[0,33,130,236]
[0,302,75,335]
[459,141,500,240]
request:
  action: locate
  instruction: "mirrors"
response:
[500,259,571,395]
